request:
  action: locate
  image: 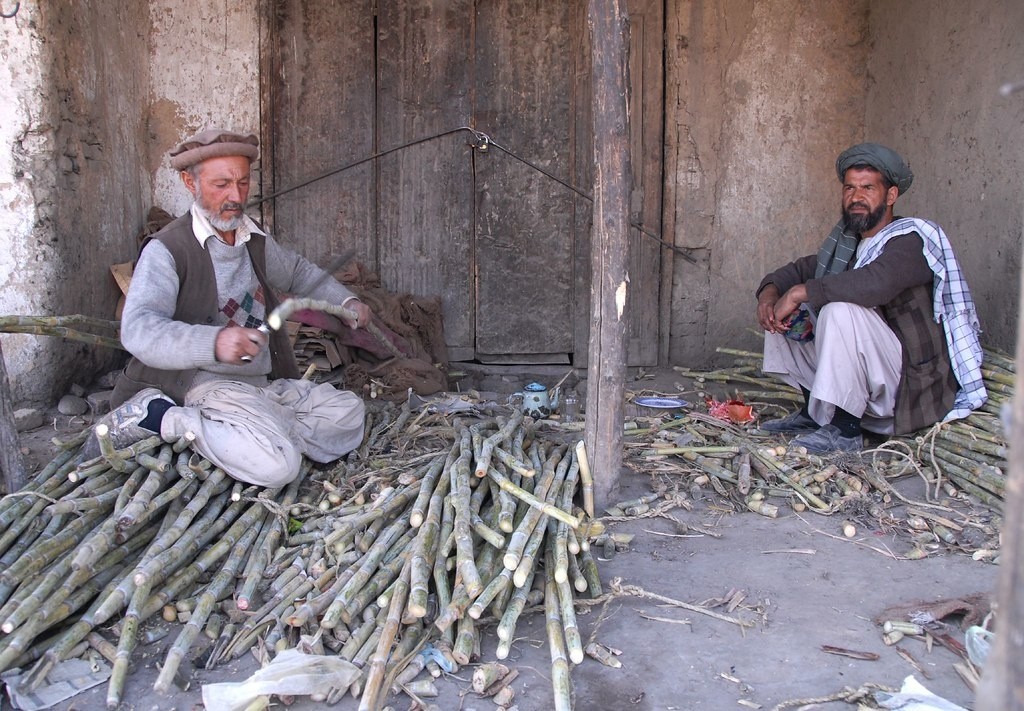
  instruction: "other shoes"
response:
[967,624,996,670]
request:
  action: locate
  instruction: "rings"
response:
[241,356,253,364]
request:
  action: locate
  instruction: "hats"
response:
[168,129,259,171]
[835,143,913,197]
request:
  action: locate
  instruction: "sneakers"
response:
[81,387,177,460]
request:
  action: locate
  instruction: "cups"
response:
[559,394,580,424]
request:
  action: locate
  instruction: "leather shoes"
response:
[791,423,864,455]
[761,410,824,433]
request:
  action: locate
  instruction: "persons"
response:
[755,143,959,459]
[84,130,374,489]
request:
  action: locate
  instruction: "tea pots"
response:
[506,383,561,420]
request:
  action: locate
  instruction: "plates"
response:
[635,396,688,409]
